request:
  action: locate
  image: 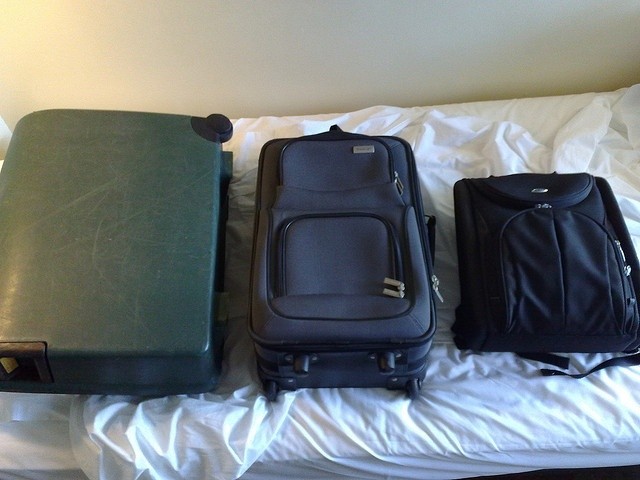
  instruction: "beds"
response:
[0,84,638,477]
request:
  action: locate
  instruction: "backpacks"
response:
[452,170,639,379]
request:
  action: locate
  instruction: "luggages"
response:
[0,109,233,394]
[248,124,444,403]
